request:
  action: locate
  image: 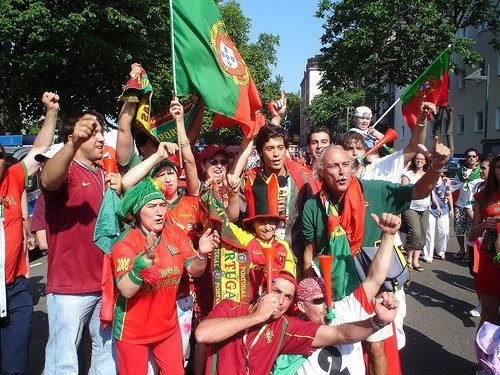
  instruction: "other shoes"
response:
[461,255,469,263]
[470,302,482,317]
[454,252,466,259]
[439,255,446,261]
[413,265,424,272]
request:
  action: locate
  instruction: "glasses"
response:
[467,155,477,158]
[209,158,228,165]
[302,297,325,305]
[0,152,6,159]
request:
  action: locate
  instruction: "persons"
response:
[0,63,449,375]
[468,148,500,335]
[401,152,454,271]
[450,148,495,316]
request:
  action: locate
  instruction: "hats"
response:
[119,178,166,214]
[148,158,178,179]
[354,106,372,118]
[293,277,325,302]
[200,145,229,162]
[34,143,64,162]
[263,269,297,289]
[241,173,287,222]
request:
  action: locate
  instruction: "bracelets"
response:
[196,250,206,260]
[416,121,427,126]
[181,140,189,145]
[373,315,384,327]
[370,317,380,330]
[181,142,190,148]
[181,139,189,143]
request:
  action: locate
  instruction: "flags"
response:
[169,0,265,138]
[400,47,449,133]
[309,214,361,302]
[93,185,121,333]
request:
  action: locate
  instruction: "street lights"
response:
[462,64,490,156]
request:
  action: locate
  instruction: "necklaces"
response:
[434,172,450,204]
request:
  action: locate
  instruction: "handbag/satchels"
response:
[355,244,411,292]
